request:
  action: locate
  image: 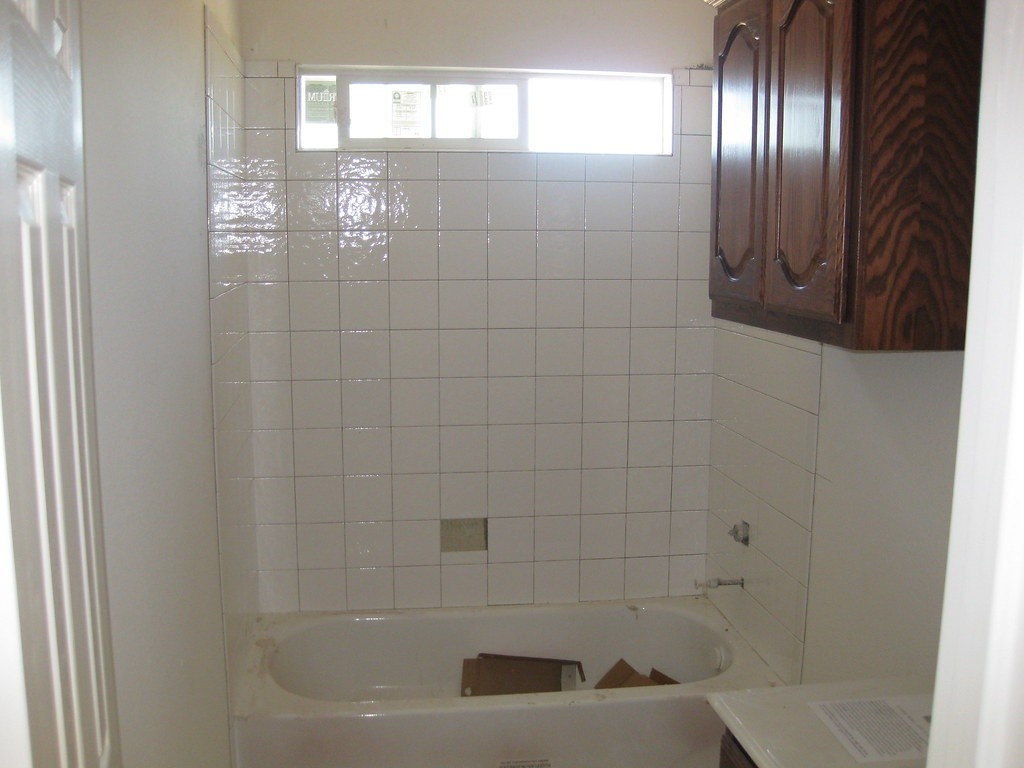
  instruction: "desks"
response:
[706,677,932,768]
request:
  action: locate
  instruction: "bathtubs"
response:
[234,593,781,768]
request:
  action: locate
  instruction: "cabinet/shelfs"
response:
[702,0,986,352]
